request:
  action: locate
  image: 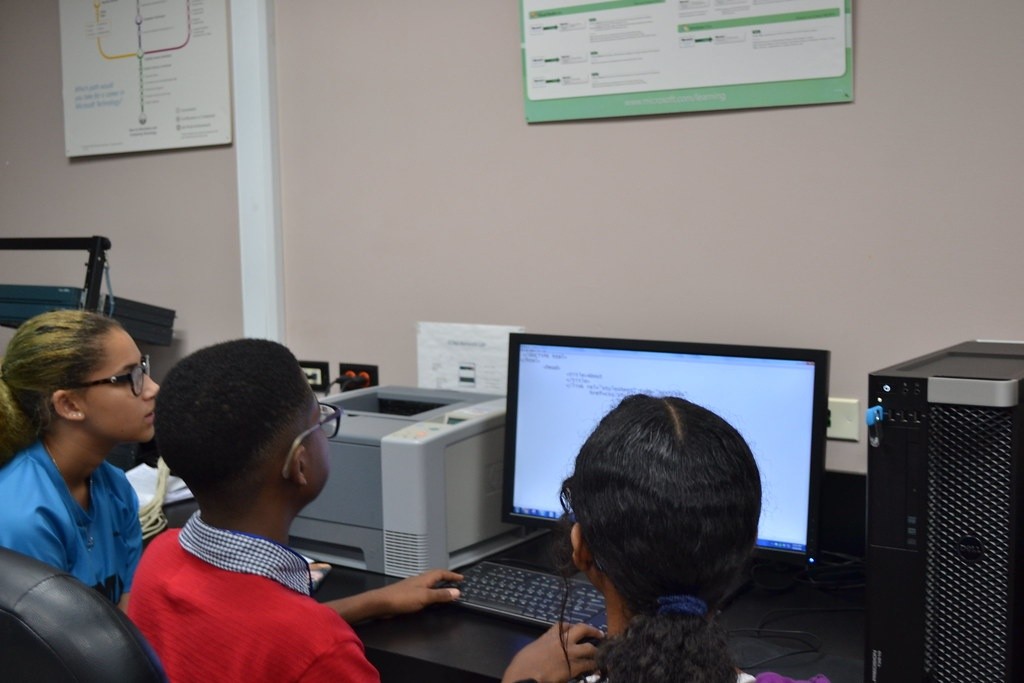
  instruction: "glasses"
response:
[70,354,150,396]
[560,490,603,571]
[282,402,342,479]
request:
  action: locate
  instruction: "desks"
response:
[151,489,863,683]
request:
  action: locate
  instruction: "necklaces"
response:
[43,442,96,552]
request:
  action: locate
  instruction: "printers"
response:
[289,384,550,578]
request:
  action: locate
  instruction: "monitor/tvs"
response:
[501,333,831,597]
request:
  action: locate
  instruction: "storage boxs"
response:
[0,283,176,346]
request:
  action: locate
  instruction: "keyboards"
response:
[439,559,608,641]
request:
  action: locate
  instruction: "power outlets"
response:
[340,362,378,393]
[297,360,329,395]
[827,399,860,441]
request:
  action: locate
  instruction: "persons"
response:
[555,393,834,683]
[1,307,162,616]
[128,337,604,683]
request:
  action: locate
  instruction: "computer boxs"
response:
[861,338,1024,683]
